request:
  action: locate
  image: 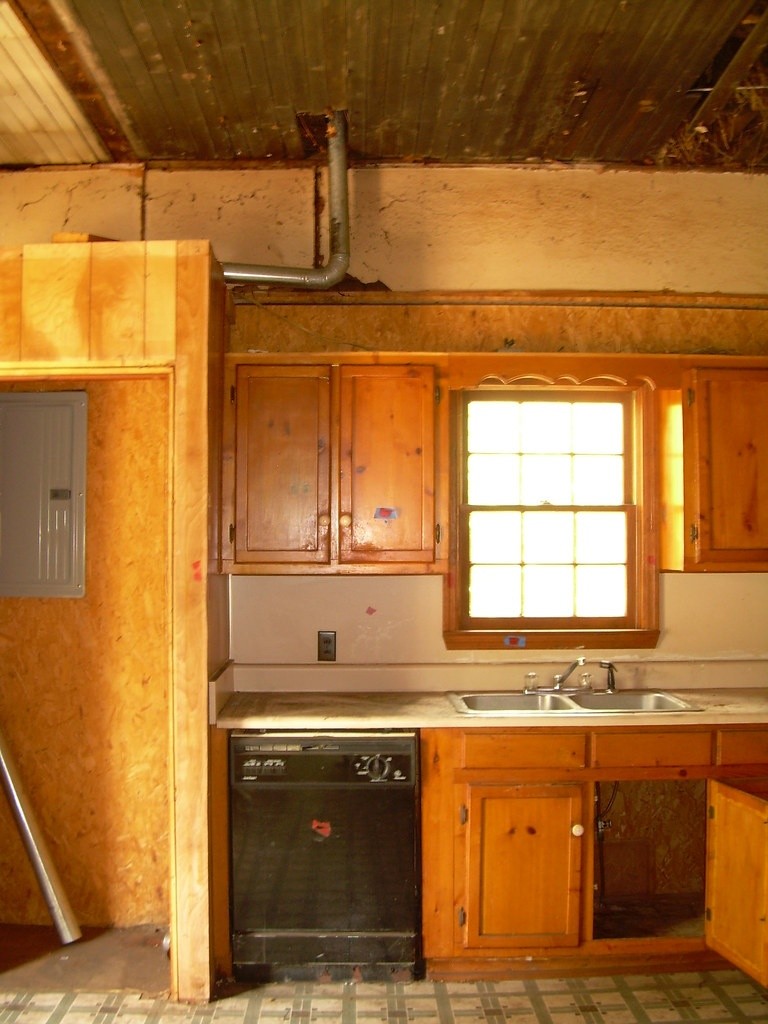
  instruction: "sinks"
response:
[443,690,578,719]
[565,687,707,719]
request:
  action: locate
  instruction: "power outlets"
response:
[317,631,337,661]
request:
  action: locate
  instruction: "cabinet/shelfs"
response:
[652,367,768,573]
[219,355,451,575]
[419,722,768,990]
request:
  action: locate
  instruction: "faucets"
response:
[598,659,621,695]
[551,655,587,692]
